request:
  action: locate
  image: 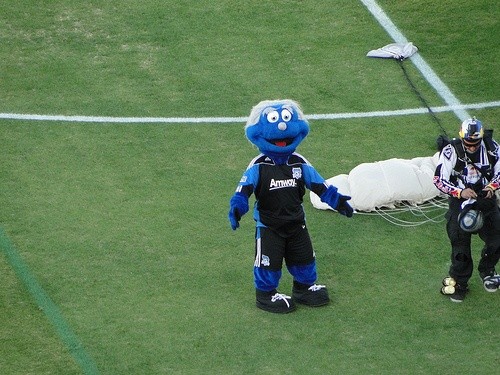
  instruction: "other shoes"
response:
[255,288,296,314]
[450,280,470,302]
[479,267,500,292]
[291,280,329,307]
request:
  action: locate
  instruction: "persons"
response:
[429,115,500,302]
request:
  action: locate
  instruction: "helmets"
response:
[459,118,483,144]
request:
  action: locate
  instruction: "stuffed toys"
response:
[229,99,354,315]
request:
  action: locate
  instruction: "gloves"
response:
[321,185,353,218]
[228,195,249,230]
